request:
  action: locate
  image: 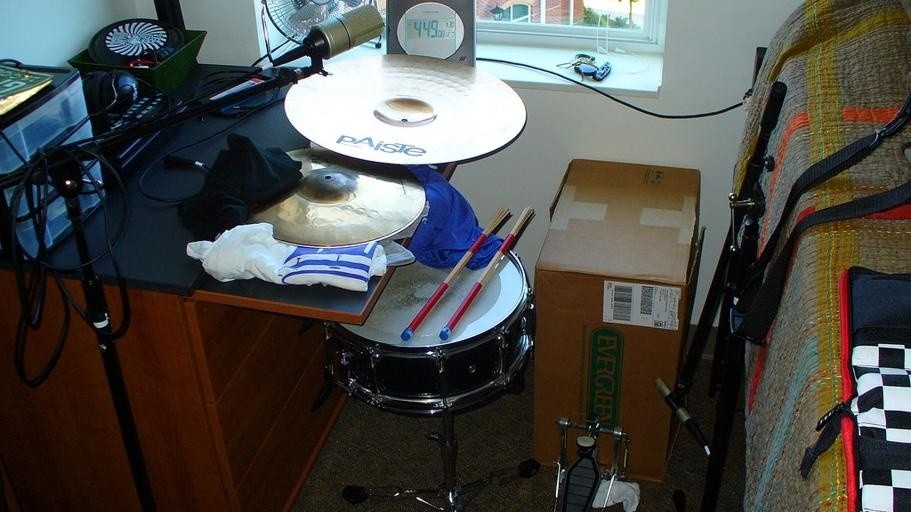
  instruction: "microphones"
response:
[654,377,709,448]
[271,5,384,67]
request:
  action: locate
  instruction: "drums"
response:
[322,221,534,416]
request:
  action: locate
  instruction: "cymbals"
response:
[249,146,426,248]
[282,50,527,168]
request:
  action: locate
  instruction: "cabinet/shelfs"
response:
[0,55,463,507]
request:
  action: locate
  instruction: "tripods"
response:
[341,412,541,512]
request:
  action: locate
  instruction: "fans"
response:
[262,0,380,44]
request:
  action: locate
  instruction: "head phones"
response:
[81,69,142,120]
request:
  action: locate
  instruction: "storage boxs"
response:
[534,157,703,480]
[3,64,101,253]
